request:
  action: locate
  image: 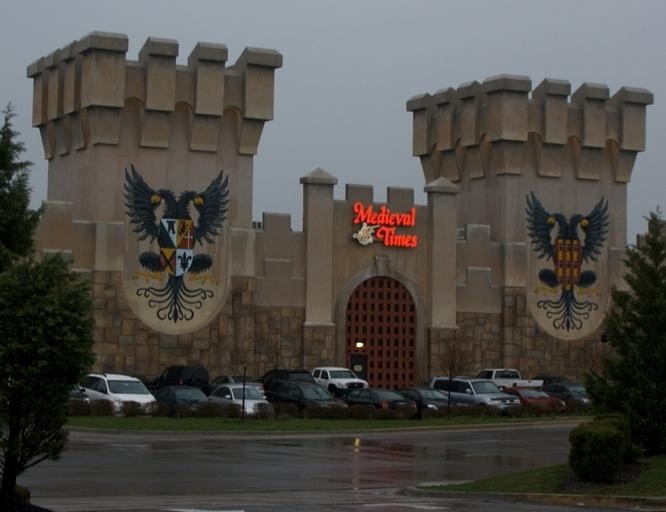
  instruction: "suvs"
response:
[429,375,520,418]
[151,364,212,398]
[311,367,369,398]
[77,371,158,417]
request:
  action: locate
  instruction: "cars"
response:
[256,369,316,388]
[207,384,269,420]
[262,380,349,419]
[395,387,462,418]
[158,386,212,417]
[341,388,418,421]
[530,376,596,413]
[67,383,91,417]
[210,374,265,395]
[502,387,567,416]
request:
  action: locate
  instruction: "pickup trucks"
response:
[472,368,544,392]
